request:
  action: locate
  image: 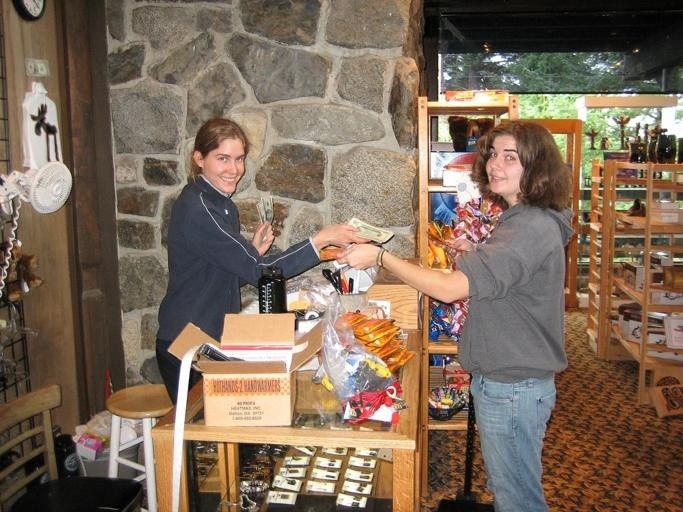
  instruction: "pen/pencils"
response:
[340,272,353,294]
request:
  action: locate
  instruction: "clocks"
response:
[13,0,49,21]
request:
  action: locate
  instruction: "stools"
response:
[104,381,178,511]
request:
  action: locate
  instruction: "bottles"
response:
[23,434,80,492]
[257,266,287,314]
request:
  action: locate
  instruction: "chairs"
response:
[0,385,142,512]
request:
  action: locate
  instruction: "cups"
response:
[628,134,683,164]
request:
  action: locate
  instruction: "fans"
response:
[0,159,77,215]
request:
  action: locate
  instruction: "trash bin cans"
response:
[54,434,139,481]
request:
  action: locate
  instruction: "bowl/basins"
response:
[339,291,369,314]
[427,388,467,421]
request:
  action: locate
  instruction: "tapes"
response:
[304,311,319,320]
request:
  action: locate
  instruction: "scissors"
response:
[322,269,342,295]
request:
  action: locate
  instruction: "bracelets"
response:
[375,248,388,268]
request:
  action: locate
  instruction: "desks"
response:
[150,281,422,512]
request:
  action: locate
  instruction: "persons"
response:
[336,121,576,512]
[155,118,372,407]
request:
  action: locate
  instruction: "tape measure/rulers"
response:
[333,269,340,289]
